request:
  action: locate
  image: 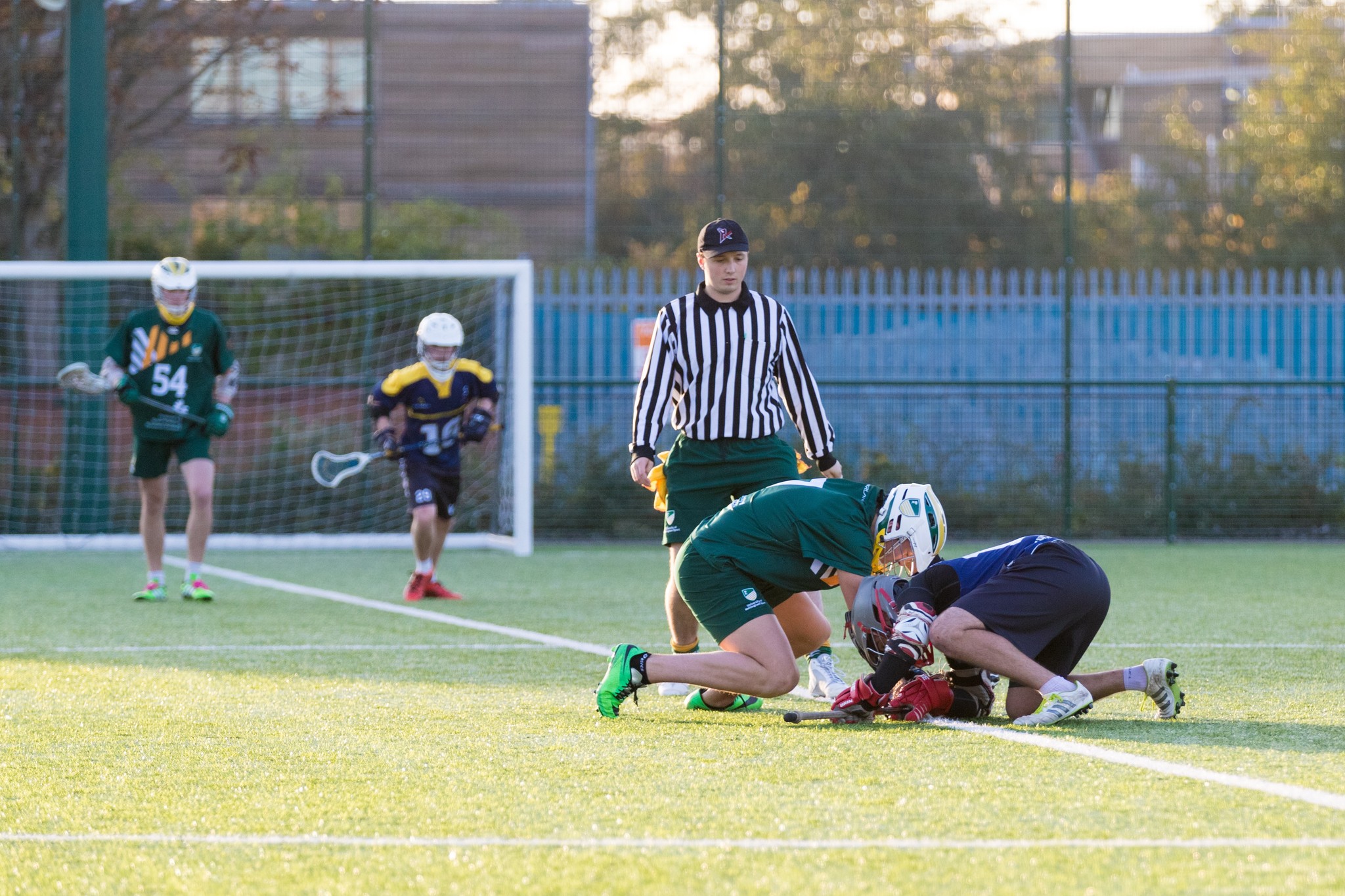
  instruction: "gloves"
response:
[371,425,406,462]
[204,400,235,438]
[109,372,142,404]
[463,407,493,442]
[887,675,954,722]
[831,673,892,724]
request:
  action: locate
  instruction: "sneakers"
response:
[1140,658,1185,719]
[658,680,689,696]
[1013,680,1094,726]
[684,687,762,713]
[421,576,464,600]
[180,572,215,600]
[402,563,434,601]
[133,577,168,602]
[594,644,648,719]
[808,653,849,699]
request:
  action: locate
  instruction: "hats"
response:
[697,218,749,258]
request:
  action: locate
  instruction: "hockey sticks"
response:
[57,362,206,426]
[783,706,912,724]
[310,423,507,489]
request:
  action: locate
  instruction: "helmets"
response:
[151,256,197,325]
[416,312,464,382]
[871,483,947,573]
[851,574,935,673]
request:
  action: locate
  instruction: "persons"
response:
[832,534,1186,727]
[99,257,242,601]
[595,477,948,720]
[366,312,501,601]
[628,218,851,701]
